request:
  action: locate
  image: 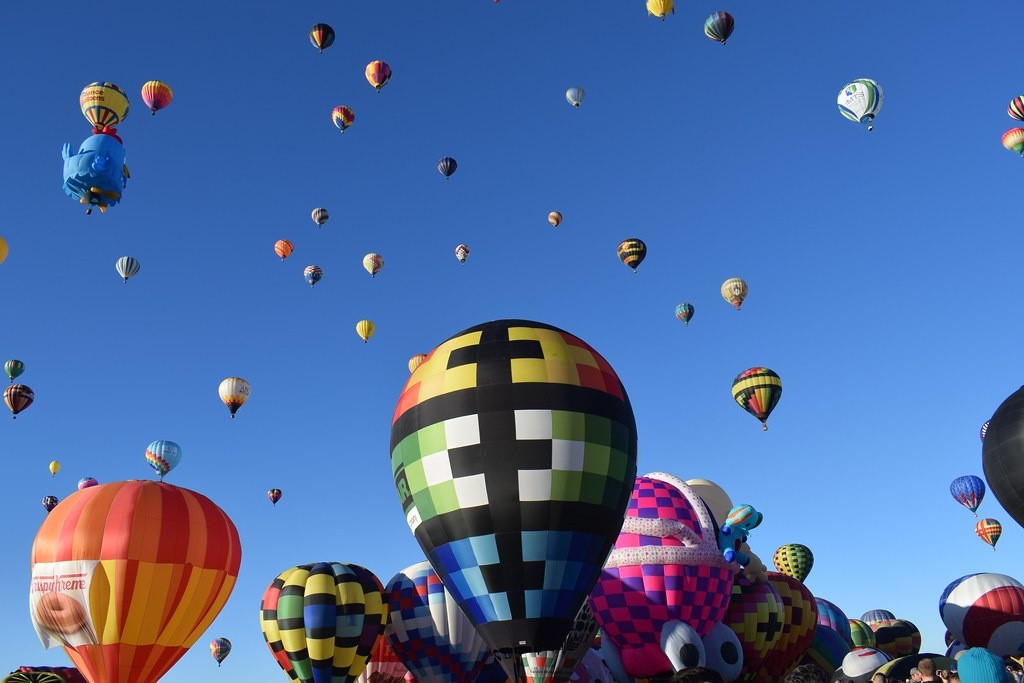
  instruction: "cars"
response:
[435,0,736,228]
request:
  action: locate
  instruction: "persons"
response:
[669,646,1023,683]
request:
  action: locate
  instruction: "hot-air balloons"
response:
[456,244,470,267]
[140,78,173,116]
[674,304,696,327]
[729,364,784,432]
[719,278,749,311]
[331,105,355,133]
[256,318,1023,683]
[616,236,648,275]
[5,359,26,382]
[837,78,1024,163]
[308,23,335,53]
[0,376,251,683]
[304,264,323,289]
[274,238,295,262]
[62,79,133,215]
[116,255,139,283]
[362,252,384,279]
[312,207,329,232]
[364,59,392,93]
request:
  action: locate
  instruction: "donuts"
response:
[35,591,87,633]
